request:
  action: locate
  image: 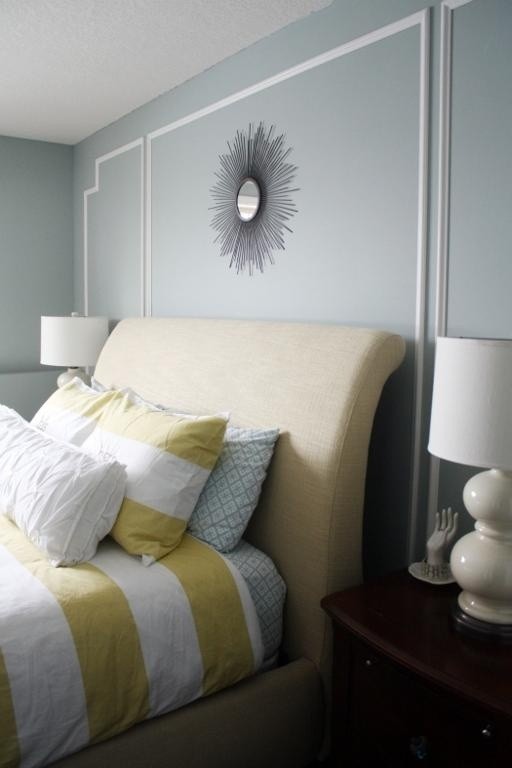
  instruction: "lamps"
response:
[40,311,109,386]
[427,336,512,638]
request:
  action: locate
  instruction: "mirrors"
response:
[235,177,261,222]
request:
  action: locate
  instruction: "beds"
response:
[0,316,407,768]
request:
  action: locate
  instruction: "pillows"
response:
[27,376,230,568]
[89,377,281,555]
[0,404,128,567]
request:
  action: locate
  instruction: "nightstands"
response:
[322,557,512,768]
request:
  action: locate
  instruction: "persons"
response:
[425,506,459,568]
